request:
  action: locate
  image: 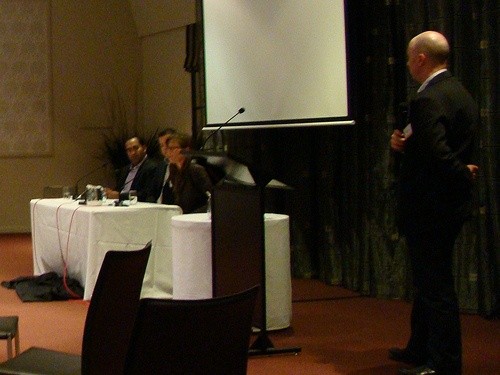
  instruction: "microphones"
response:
[398,103,408,130]
[200,108,245,152]
[74,162,149,205]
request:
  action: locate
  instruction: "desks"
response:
[171,212,292,332]
[30,198,182,302]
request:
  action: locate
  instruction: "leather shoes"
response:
[388,349,430,366]
[398,365,440,375]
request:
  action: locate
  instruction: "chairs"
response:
[0,239,260,375]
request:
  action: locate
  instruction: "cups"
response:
[63,186,69,200]
[129,190,137,205]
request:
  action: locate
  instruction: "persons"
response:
[387,31,480,375]
[104,128,176,204]
[165,134,211,214]
